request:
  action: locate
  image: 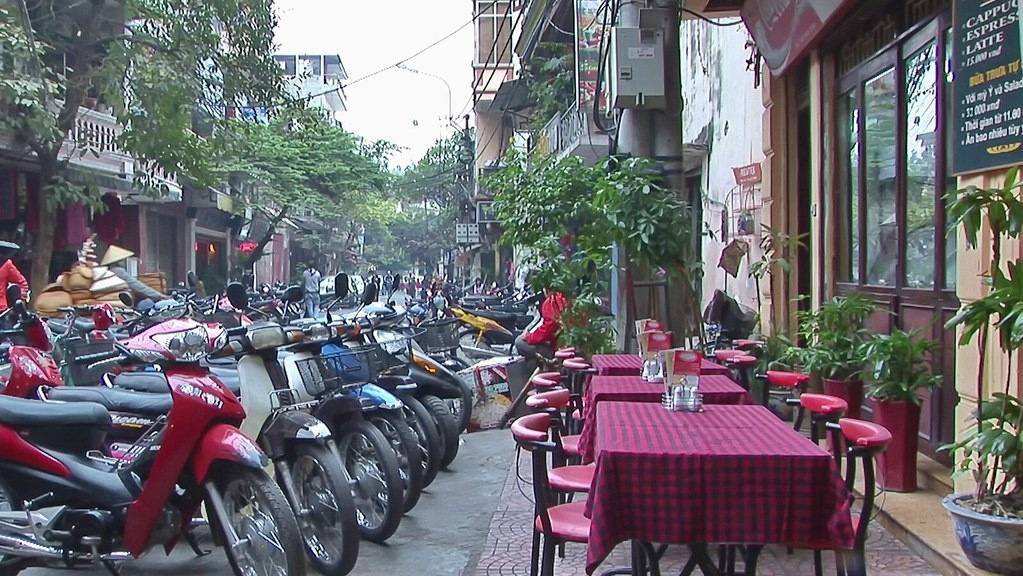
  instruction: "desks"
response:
[578,376,752,465]
[584,402,855,576]
[590,354,729,375]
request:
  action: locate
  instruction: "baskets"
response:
[703,289,758,339]
[294,326,428,396]
[423,317,460,352]
[66,338,114,386]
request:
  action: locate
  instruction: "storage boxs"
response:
[65,338,122,388]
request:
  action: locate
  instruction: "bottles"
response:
[737,209,755,235]
[671,378,700,412]
[641,354,662,380]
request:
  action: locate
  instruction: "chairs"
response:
[512,348,892,576]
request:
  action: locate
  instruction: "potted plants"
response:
[856,316,944,493]
[776,292,827,440]
[939,163,1023,574]
[814,289,899,455]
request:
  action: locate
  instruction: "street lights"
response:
[397,63,452,148]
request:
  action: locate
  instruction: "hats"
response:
[100,244,134,265]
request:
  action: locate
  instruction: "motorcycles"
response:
[0,270,552,576]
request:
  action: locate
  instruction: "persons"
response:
[488,279,499,296]
[100,245,173,304]
[301,261,324,319]
[514,287,574,360]
[504,258,517,284]
[468,278,483,292]
[383,271,432,300]
[369,273,381,303]
